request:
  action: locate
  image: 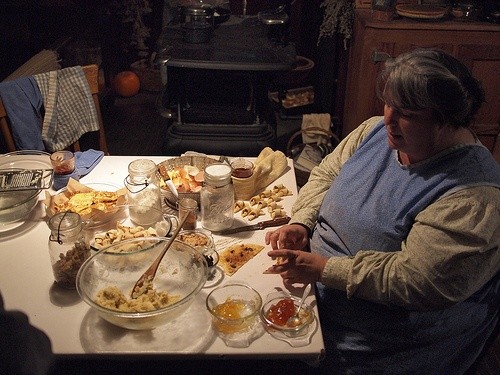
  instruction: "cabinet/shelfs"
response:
[338,3,500,164]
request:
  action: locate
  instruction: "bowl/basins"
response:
[180,4,214,44]
[179,247,220,279]
[80,204,171,271]
[76,237,208,330]
[257,10,289,37]
[170,229,215,279]
[273,56,314,85]
[155,156,222,205]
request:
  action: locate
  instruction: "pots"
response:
[170,5,231,25]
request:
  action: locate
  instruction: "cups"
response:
[230,172,256,200]
[51,151,75,175]
[230,158,254,178]
[204,282,262,342]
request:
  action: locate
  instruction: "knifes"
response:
[157,165,178,199]
[221,216,292,236]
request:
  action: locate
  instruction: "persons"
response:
[0,288,55,375]
[265,48,500,375]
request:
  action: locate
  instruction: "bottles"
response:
[124,158,163,226]
[178,198,198,230]
[45,210,93,288]
[200,163,234,231]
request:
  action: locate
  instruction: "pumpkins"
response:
[113,71,140,97]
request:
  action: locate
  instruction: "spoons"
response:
[131,211,190,301]
[287,283,312,328]
[259,292,317,347]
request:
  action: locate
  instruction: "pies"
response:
[218,243,266,276]
[60,192,119,217]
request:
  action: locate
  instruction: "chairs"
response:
[0,65,109,157]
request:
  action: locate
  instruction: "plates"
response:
[0,150,51,209]
[395,4,446,19]
[0,194,40,233]
[44,181,126,229]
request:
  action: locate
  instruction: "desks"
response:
[153,7,297,160]
[0,150,325,375]
